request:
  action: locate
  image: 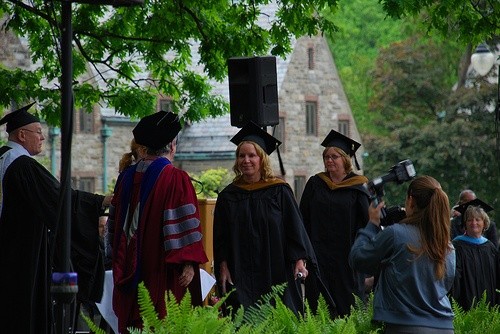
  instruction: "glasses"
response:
[20,128,42,134]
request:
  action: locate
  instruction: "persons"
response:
[119,137,143,172]
[349,175,456,334]
[451,190,498,248]
[299,129,374,320]
[111,110,209,334]
[213,125,338,320]
[451,198,500,314]
[99,216,112,271]
[0,102,114,334]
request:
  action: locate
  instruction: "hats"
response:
[453,198,494,215]
[132,111,183,149]
[230,120,286,176]
[321,128,364,170]
[0,101,41,132]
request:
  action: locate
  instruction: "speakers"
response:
[228,56,279,128]
